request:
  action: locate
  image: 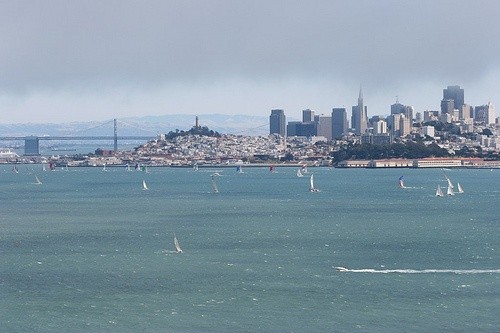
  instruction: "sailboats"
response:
[235,163,246,175]
[172,232,185,255]
[399,176,407,189]
[308,174,319,193]
[141,178,149,192]
[192,161,199,174]
[433,177,464,197]
[10,158,70,186]
[101,162,150,174]
[295,165,307,178]
[208,173,221,195]
[269,163,279,174]
